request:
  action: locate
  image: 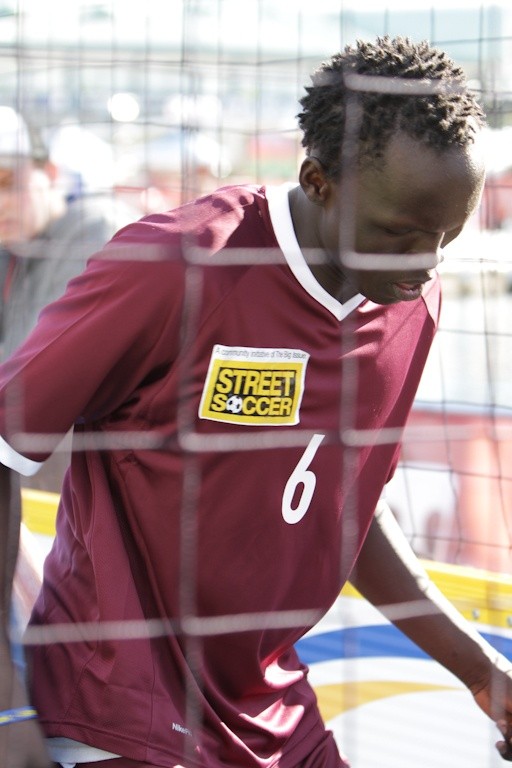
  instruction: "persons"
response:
[0,34,511,768]
[0,72,512,768]
[0,103,119,498]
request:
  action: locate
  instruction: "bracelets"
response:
[0,705,38,726]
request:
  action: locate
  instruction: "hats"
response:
[0,106,49,160]
